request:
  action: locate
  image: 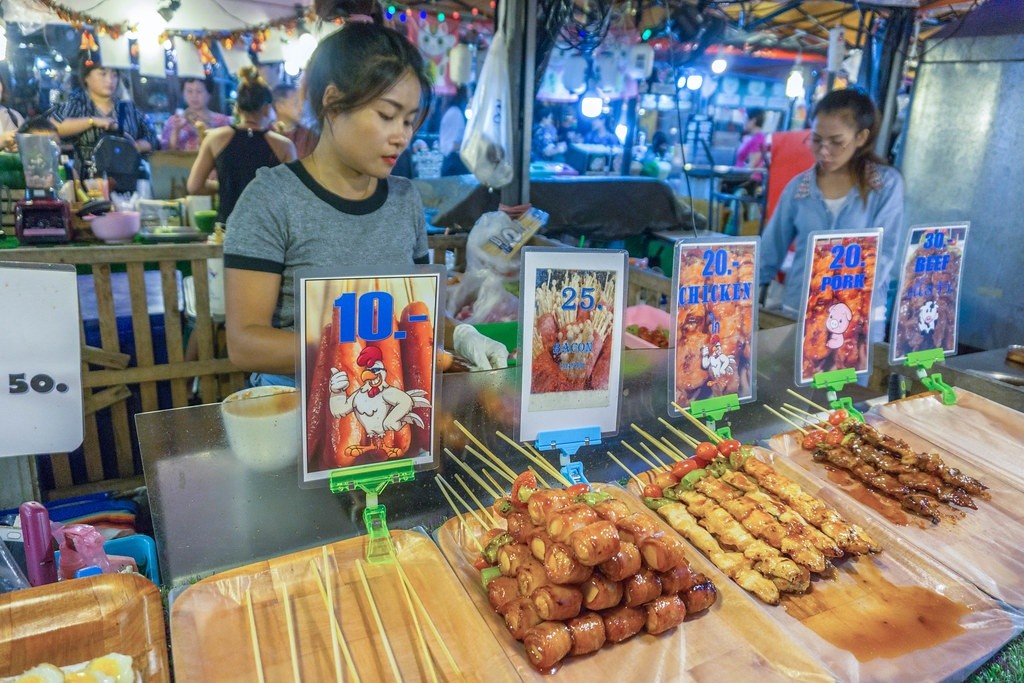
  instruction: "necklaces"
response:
[248,128,253,137]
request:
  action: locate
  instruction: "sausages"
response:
[306,300,433,469]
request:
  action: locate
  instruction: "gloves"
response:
[453,323,508,370]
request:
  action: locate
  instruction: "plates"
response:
[139,226,206,237]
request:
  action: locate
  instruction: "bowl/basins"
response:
[90,212,140,243]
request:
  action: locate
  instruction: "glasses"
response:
[802,130,861,152]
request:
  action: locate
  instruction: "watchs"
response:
[89,116,94,127]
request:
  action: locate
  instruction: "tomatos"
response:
[644,439,742,498]
[474,470,589,569]
[803,409,847,449]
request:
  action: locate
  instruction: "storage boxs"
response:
[53,534,160,586]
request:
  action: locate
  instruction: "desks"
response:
[183,274,226,398]
[653,228,731,241]
[413,172,708,238]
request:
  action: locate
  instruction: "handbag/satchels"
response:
[460,0,513,188]
[90,128,140,191]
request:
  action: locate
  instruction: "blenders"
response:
[14,133,72,242]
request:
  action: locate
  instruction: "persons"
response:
[268,83,319,159]
[643,131,667,164]
[17,119,59,139]
[163,74,228,151]
[50,63,163,154]
[0,75,25,151]
[729,106,766,195]
[441,82,476,177]
[184,67,298,402]
[531,107,620,164]
[222,0,508,387]
[760,89,904,389]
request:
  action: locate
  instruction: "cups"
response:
[195,209,218,232]
[222,384,301,469]
